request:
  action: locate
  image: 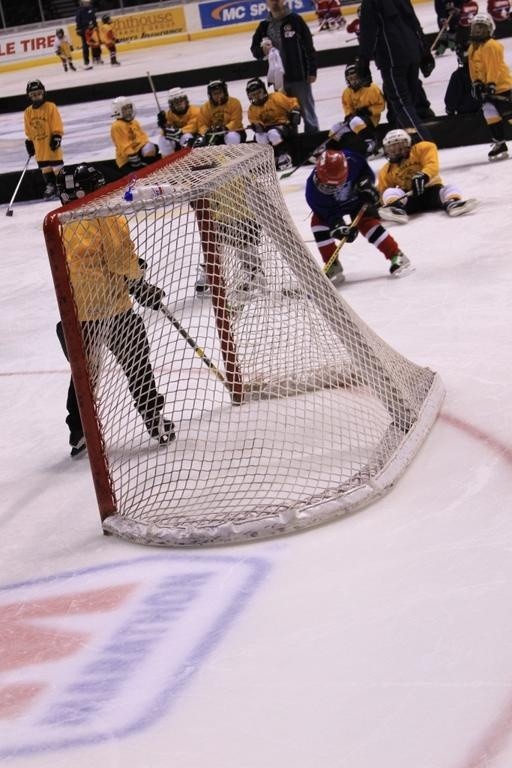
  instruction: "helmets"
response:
[206,80,228,103]
[313,150,349,195]
[382,129,410,149]
[27,79,47,105]
[112,96,134,121]
[166,87,188,114]
[470,12,497,36]
[245,79,267,96]
[344,59,365,78]
[56,164,104,204]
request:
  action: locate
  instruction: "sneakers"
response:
[238,264,269,292]
[378,205,408,224]
[486,137,508,157]
[387,251,411,275]
[277,152,290,165]
[43,183,55,198]
[110,60,120,65]
[65,439,90,458]
[191,264,214,292]
[83,63,93,70]
[442,199,474,218]
[322,263,343,281]
[93,57,104,64]
[141,414,175,439]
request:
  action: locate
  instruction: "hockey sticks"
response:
[6,155,31,217]
[161,306,266,391]
[281,203,369,300]
[280,119,349,180]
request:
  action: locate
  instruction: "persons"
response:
[305,149,413,287]
[382,78,434,124]
[455,0,479,67]
[251,0,319,136]
[24,80,64,202]
[193,82,245,148]
[445,65,484,118]
[308,57,385,164]
[192,152,269,298]
[56,162,175,457]
[75,0,98,70]
[486,0,511,39]
[54,29,76,72]
[434,1,462,58]
[377,128,478,224]
[86,21,104,64]
[110,97,162,174]
[468,13,512,161]
[356,0,435,144]
[101,14,119,65]
[346,5,362,34]
[313,0,346,31]
[157,87,198,160]
[245,78,301,170]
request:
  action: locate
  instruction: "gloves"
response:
[166,127,182,139]
[129,280,165,313]
[359,188,379,208]
[50,134,61,151]
[157,111,167,129]
[329,221,357,243]
[24,139,36,157]
[129,153,139,168]
[254,122,265,133]
[410,171,429,197]
[206,126,225,147]
[418,45,435,77]
[471,80,485,103]
[283,122,297,135]
[487,83,498,103]
